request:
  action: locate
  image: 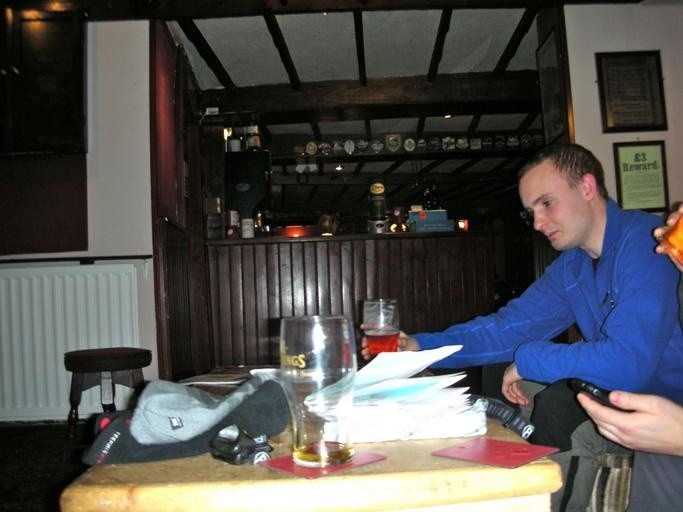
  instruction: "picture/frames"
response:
[612,140,669,213]
[593,49,669,133]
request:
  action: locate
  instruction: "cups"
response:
[660,212,682,274]
[229,137,242,150]
[246,125,262,151]
[277,313,357,468]
[362,298,400,356]
[227,210,256,239]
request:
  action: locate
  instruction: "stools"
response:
[586,453,631,511]
[64,348,151,431]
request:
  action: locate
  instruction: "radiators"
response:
[0,264,139,421]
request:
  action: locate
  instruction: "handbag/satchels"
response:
[84,374,292,466]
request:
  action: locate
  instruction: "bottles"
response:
[393,206,405,231]
[294,129,544,159]
[368,176,387,234]
[318,213,333,236]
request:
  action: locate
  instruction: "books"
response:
[303,342,487,442]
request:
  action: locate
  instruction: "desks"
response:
[60,373,564,511]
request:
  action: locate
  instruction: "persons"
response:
[576,198,683,511]
[357,139,683,511]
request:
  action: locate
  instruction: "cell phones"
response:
[568,378,631,412]
